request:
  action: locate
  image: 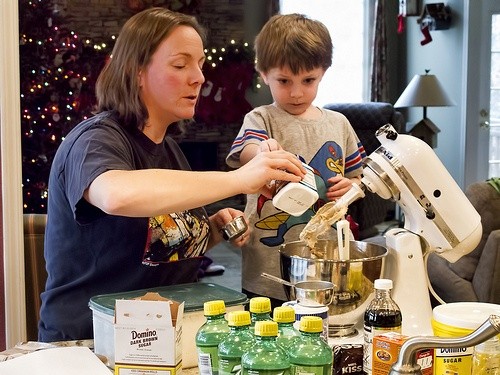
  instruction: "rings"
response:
[241,234,245,239]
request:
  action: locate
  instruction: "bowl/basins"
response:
[219,216,248,242]
[278,239,389,316]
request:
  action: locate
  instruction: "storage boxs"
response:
[88,283,250,375]
[372,333,434,375]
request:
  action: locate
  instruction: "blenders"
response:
[278,122,483,375]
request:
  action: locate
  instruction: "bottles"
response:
[286,316,333,375]
[273,306,302,346]
[241,321,291,375]
[362,278,402,375]
[272,154,319,217]
[249,296,275,327]
[217,311,256,375]
[195,300,229,375]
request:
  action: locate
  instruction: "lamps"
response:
[394,69,456,119]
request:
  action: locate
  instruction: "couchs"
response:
[323,102,406,229]
[428,180,500,309]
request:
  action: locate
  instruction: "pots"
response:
[260,272,336,305]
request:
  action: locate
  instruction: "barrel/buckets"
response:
[432,302,500,375]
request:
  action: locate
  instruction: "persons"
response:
[38,7,307,342]
[226,15,367,312]
